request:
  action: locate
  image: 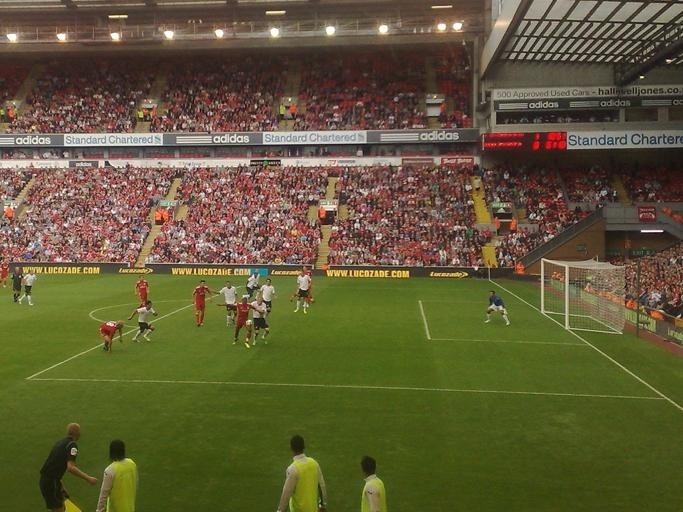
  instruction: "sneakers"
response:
[294,310,299,313]
[29,304,33,305]
[232,335,267,348]
[18,299,21,305]
[505,322,511,325]
[131,338,139,343]
[485,318,492,324]
[143,336,150,341]
[303,309,307,314]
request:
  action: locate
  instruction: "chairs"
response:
[584,242,682,326]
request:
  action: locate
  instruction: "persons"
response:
[35,420,99,512]
[128,300,158,343]
[275,433,329,512]
[1,68,683,268]
[98,320,125,352]
[470,260,488,279]
[135,275,150,308]
[511,261,683,326]
[485,291,511,325]
[1,262,38,306]
[94,438,139,512]
[192,271,276,349]
[358,454,389,512]
[290,266,314,314]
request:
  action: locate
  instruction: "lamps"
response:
[3,8,469,48]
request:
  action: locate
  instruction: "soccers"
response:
[246,320,253,327]
